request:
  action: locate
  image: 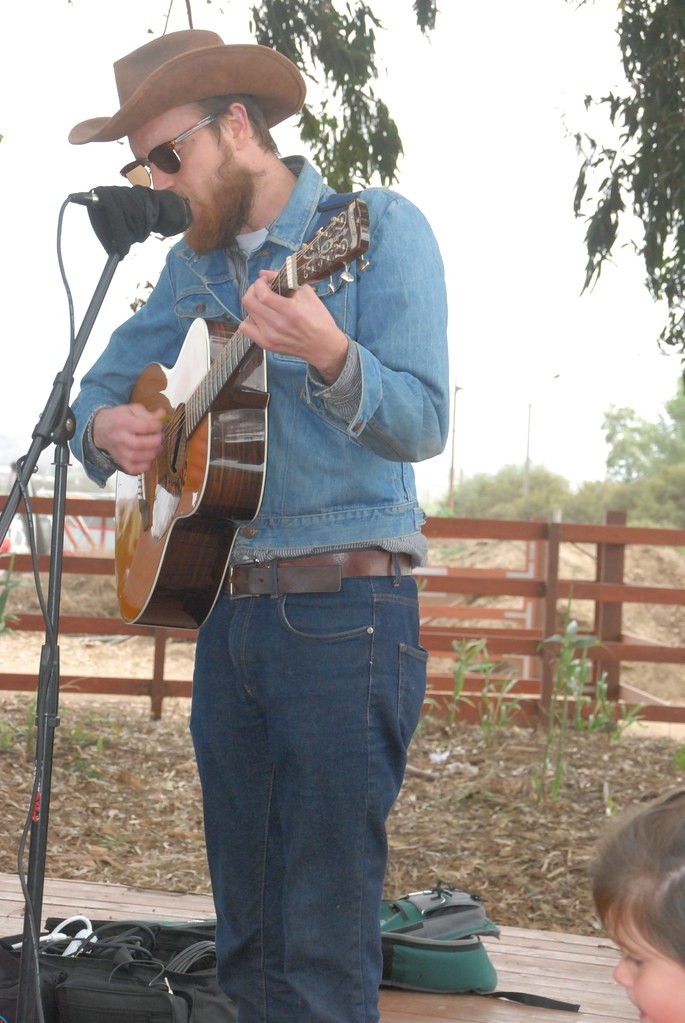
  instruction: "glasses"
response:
[119,107,228,187]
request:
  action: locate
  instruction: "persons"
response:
[68,30,450,1023]
[591,788,685,1023]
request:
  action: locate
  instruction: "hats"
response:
[68,29,307,146]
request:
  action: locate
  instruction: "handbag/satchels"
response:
[0,916,238,1023]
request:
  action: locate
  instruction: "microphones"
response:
[67,189,193,237]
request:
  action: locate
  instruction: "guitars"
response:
[112,197,372,632]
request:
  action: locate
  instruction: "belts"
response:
[222,548,414,597]
